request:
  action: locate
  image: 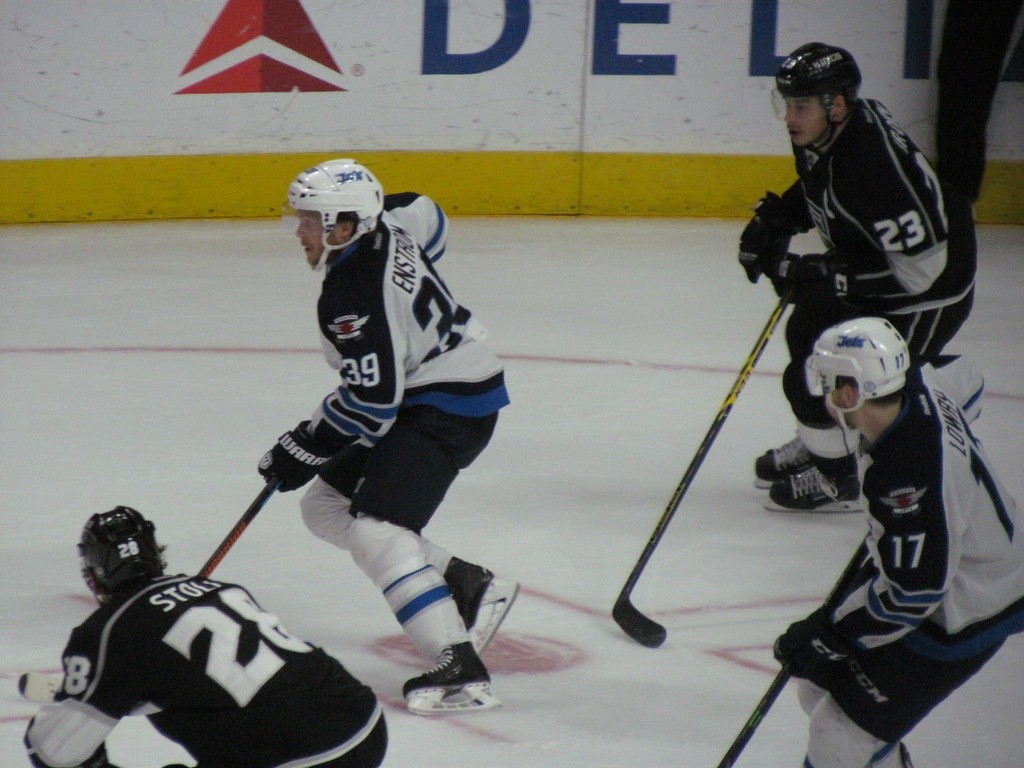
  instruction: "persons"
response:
[740,42,975,512]
[259,159,522,712]
[772,317,1024,768]
[22,507,389,768]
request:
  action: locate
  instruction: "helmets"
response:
[79,506,166,597]
[775,42,861,110]
[815,317,910,400]
[287,158,384,235]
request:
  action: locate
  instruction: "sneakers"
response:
[445,558,520,655]
[763,453,863,513]
[402,642,500,716]
[755,436,814,489]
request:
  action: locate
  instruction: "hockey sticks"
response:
[609,277,803,650]
[716,530,873,768]
[17,474,283,705]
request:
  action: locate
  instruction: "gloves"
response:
[740,191,810,283]
[257,420,341,494]
[767,252,855,307]
[773,605,857,681]
